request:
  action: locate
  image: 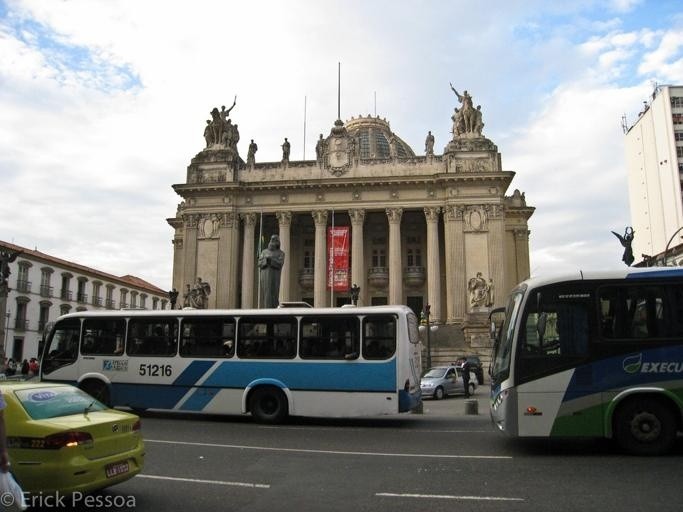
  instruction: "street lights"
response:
[166,288,180,310]
[425,302,433,370]
[349,283,361,309]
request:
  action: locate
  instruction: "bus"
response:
[484,263,683,460]
[36,299,423,425]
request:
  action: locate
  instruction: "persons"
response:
[183,277,210,308]
[622,225,635,269]
[0,390,11,511]
[203,103,240,149]
[485,279,495,308]
[450,82,484,135]
[59,334,80,360]
[257,234,285,307]
[2,355,38,380]
[82,326,393,361]
[468,272,486,309]
[543,322,561,360]
[458,355,472,400]
[424,131,435,154]
[282,137,290,159]
[246,139,257,167]
[315,134,325,160]
[420,310,425,320]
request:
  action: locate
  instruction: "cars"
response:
[450,355,487,385]
[0,378,150,502]
[420,364,478,399]
